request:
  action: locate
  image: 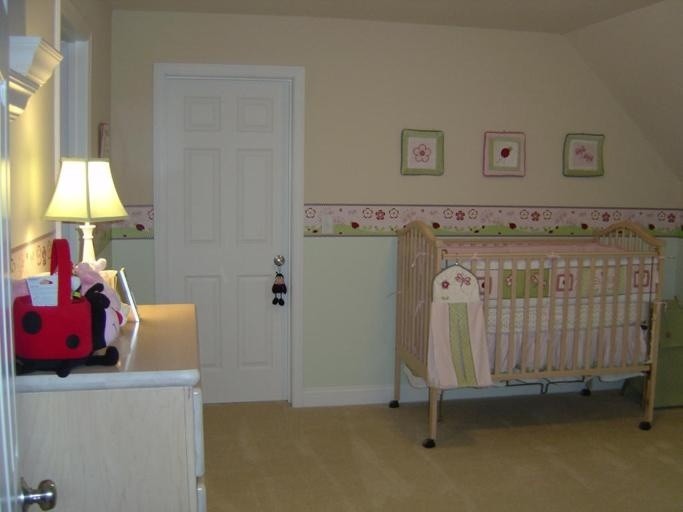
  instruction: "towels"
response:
[427,301,492,389]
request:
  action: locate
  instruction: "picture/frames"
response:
[482,132,527,178]
[400,128,444,177]
[561,132,606,178]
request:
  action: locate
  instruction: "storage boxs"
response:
[620,299,683,409]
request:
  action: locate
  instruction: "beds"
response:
[388,218,663,451]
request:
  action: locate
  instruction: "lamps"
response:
[43,157,130,276]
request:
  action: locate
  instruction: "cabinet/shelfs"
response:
[6,302,207,512]
